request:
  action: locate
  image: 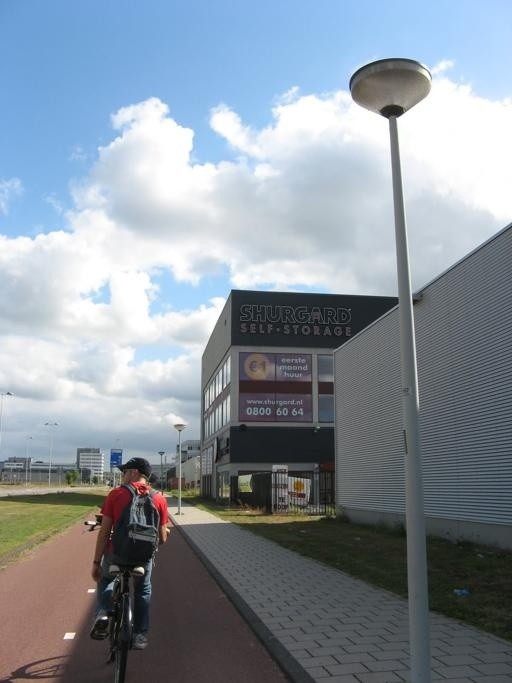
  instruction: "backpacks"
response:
[109,482,162,565]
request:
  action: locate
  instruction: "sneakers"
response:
[89,615,110,640]
[133,634,150,650]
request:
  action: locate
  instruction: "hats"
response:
[117,457,152,477]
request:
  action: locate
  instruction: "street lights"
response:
[25,436,33,488]
[44,422,59,487]
[0,392,14,432]
[349,57,431,683]
[158,452,165,496]
[173,424,187,515]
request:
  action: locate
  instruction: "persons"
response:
[91,456,170,651]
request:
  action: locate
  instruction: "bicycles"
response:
[84,514,145,683]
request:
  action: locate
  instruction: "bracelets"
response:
[93,560,102,564]
